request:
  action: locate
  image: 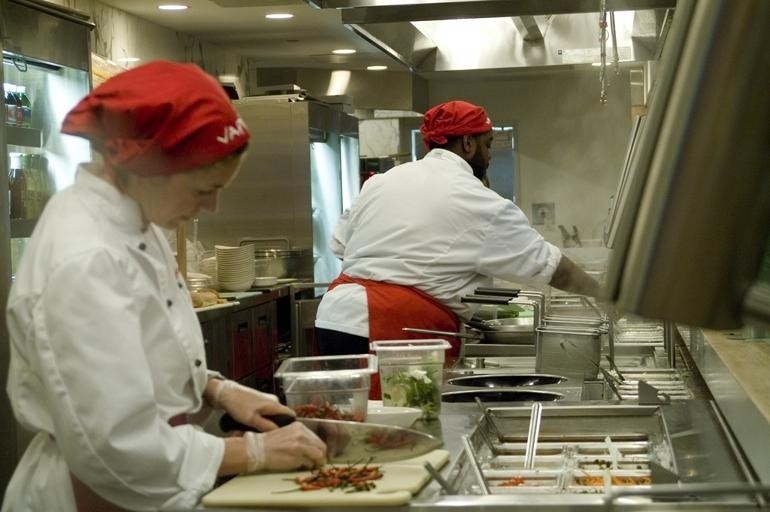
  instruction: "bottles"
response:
[10,151,49,221]
[4,82,32,128]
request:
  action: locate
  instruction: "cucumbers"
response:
[474,310,519,319]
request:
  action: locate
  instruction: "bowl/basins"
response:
[255,246,307,280]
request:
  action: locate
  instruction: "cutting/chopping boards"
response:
[201,449,451,507]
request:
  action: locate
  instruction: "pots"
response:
[400,316,536,344]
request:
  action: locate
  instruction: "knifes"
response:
[217,411,445,464]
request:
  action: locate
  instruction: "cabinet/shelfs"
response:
[198,311,229,379]
[229,298,276,396]
[277,280,333,375]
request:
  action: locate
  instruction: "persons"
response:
[307,95,611,402]
[2,57,333,510]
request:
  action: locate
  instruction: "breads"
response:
[191,292,217,307]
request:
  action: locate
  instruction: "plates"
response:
[252,276,278,287]
[188,241,255,293]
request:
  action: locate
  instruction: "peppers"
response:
[294,396,417,491]
